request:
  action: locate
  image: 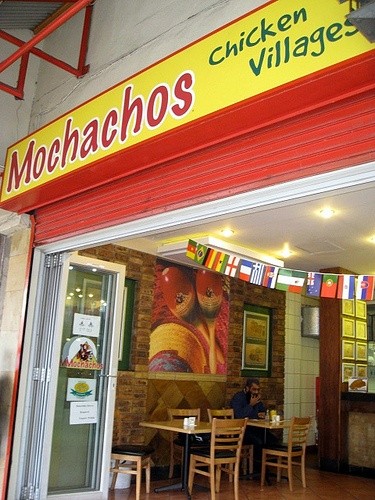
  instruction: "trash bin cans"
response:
[110,459,132,490]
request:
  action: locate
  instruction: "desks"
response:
[246,418,311,486]
[139,420,243,500]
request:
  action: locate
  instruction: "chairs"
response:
[259,416,312,490]
[109,445,155,500]
[166,407,200,479]
[207,408,253,482]
[187,417,248,500]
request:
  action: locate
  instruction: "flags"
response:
[275,268,293,291]
[356,274,375,301]
[306,272,323,296]
[249,262,266,284]
[238,259,254,281]
[186,238,230,273]
[261,265,280,289]
[336,274,356,300]
[321,273,338,298]
[224,255,241,277]
[288,270,307,293]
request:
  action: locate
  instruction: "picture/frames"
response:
[355,363,367,379]
[81,278,128,362]
[341,318,355,338]
[354,300,367,319]
[342,299,355,316]
[241,311,270,370]
[355,320,367,340]
[355,341,368,361]
[341,340,355,360]
[342,363,356,383]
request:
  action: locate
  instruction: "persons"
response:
[228,378,278,476]
